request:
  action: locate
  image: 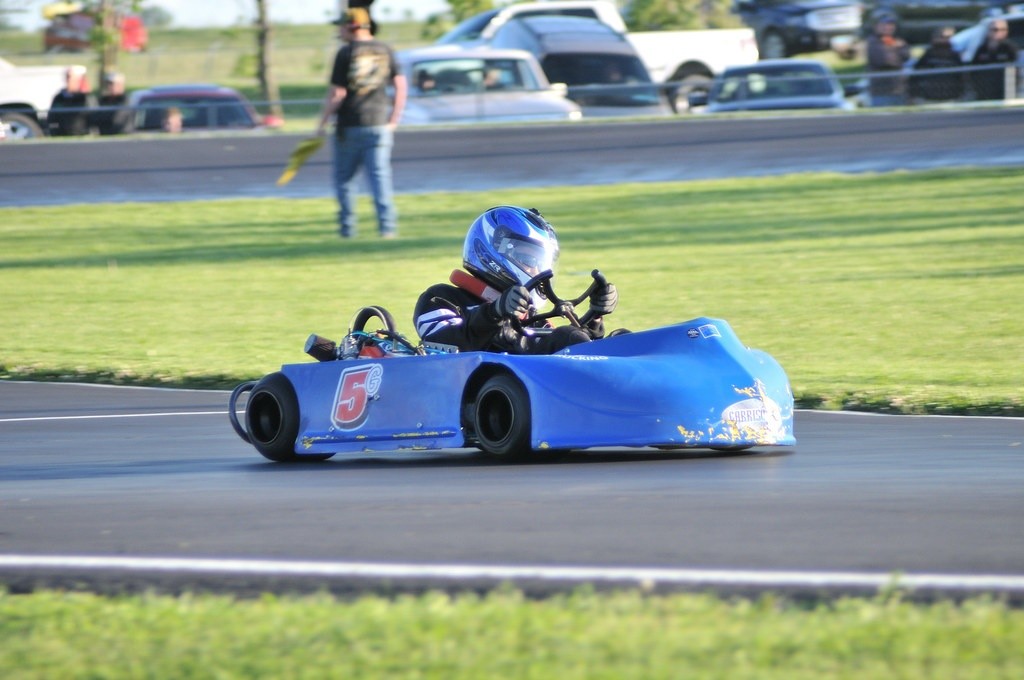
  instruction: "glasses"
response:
[940,35,950,39]
[513,251,547,273]
[991,28,1007,31]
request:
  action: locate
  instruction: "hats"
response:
[877,15,896,23]
[333,7,370,25]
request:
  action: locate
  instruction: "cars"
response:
[434,2,657,125]
[395,45,579,138]
[2,54,87,141]
[106,88,270,140]
[707,60,840,121]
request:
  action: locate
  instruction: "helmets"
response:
[463,205,560,293]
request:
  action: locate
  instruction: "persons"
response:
[864,8,1018,107]
[158,107,185,132]
[477,66,505,93]
[48,64,127,137]
[412,205,618,356]
[315,7,408,241]
[418,70,439,92]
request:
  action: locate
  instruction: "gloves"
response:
[490,285,533,321]
[590,283,618,314]
